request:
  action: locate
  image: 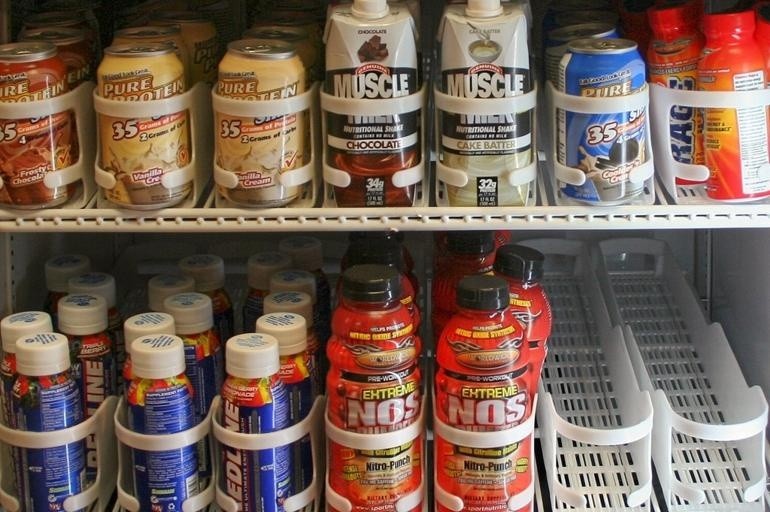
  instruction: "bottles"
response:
[324,234,431,512]
[0,233,118,512]
[432,233,551,512]
[620,4,770,207]
[118,233,232,512]
[215,232,324,512]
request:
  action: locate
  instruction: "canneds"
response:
[98,42,193,211]
[0,41,73,208]
[19,26,93,190]
[557,36,646,206]
[543,20,619,92]
[148,9,223,81]
[216,37,306,208]
[111,25,192,84]
[240,25,316,199]
